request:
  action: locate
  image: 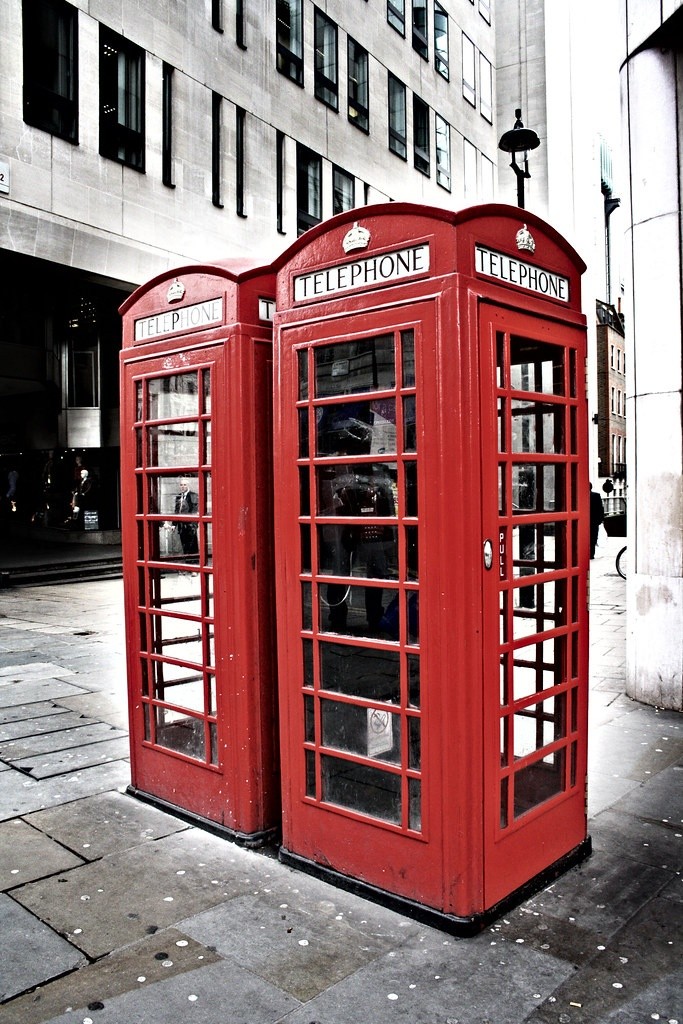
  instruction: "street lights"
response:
[498,108,541,609]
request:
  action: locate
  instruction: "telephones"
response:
[335,482,399,557]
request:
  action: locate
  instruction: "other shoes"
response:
[160,576,166,579]
[191,572,197,576]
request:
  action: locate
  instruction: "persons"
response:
[36,455,92,528]
[150,479,199,579]
[589,481,605,559]
[313,416,398,641]
